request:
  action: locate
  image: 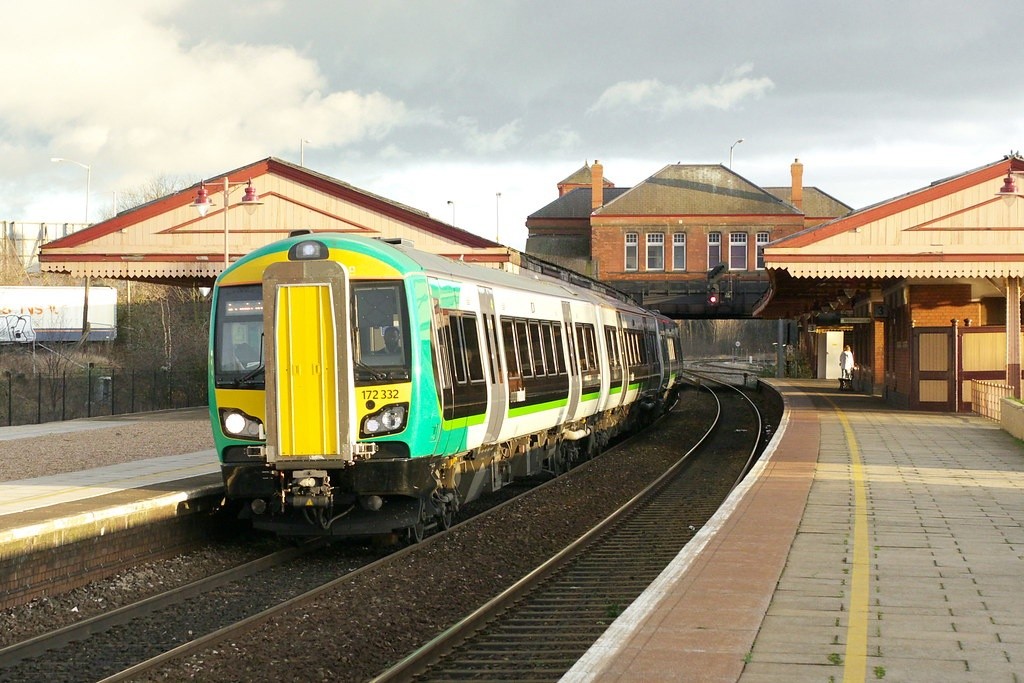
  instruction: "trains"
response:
[207,232,684,548]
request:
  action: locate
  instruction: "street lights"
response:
[729,139,744,170]
[299,138,310,167]
[190,175,266,270]
[446,200,455,228]
[49,157,92,228]
[496,192,501,243]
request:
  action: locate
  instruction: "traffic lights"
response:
[707,284,720,308]
[708,262,728,283]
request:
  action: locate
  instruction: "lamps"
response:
[794,281,857,322]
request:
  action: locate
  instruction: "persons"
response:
[840,345,854,385]
[373,327,403,354]
